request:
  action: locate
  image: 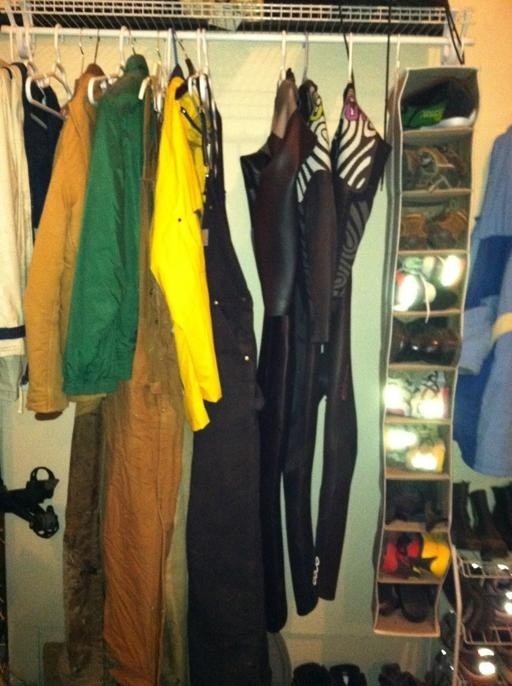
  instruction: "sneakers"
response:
[292,663,367,685]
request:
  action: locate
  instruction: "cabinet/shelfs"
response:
[372,65,479,638]
[441,545,512,686]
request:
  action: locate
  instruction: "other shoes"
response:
[378,78,512,686]
